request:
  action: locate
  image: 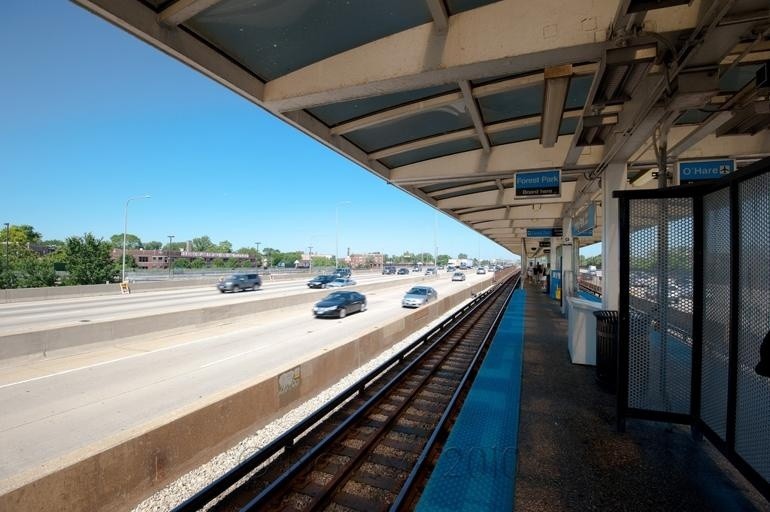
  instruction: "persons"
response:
[525,260,550,289]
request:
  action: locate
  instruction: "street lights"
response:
[122,193,352,285]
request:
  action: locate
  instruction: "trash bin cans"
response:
[593,310,648,395]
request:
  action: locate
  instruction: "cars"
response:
[578,264,712,305]
[217,260,514,319]
